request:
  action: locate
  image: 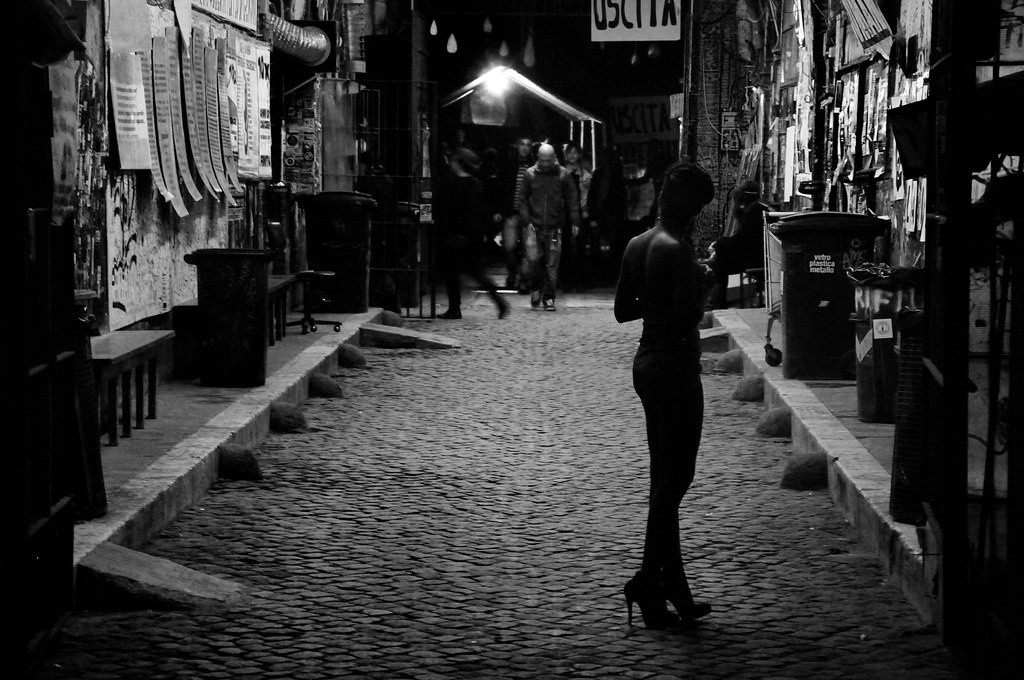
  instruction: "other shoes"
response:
[437,308,462,319]
[543,299,555,311]
[531,289,541,308]
[498,302,510,318]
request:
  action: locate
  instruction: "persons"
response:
[428,127,631,319]
[613,161,729,631]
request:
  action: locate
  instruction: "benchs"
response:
[268,274,297,347]
[91,330,175,447]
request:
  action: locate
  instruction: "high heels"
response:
[624,580,679,629]
[663,581,712,620]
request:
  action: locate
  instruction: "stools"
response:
[286,269,342,335]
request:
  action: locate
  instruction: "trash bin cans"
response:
[181,245,274,388]
[772,207,892,383]
[292,187,379,310]
